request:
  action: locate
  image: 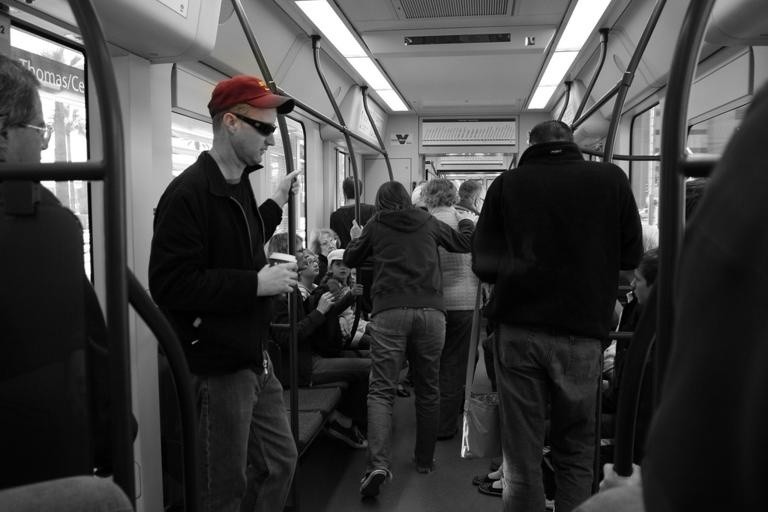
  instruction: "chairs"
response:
[309,379,348,389]
[271,412,325,455]
[283,386,342,414]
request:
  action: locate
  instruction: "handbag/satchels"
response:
[461,391,502,460]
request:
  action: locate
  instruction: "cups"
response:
[269,253,297,297]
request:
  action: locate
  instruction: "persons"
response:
[149,75,298,510]
[645,81,768,510]
[1,55,137,489]
[267,178,485,498]
[469,120,658,511]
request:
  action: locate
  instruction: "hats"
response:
[326,249,353,271]
[208,75,295,114]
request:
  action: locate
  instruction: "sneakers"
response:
[329,419,367,448]
[360,468,388,499]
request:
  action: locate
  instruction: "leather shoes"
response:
[398,385,410,397]
[473,472,504,495]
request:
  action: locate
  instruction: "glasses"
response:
[24,124,52,150]
[234,113,277,136]
